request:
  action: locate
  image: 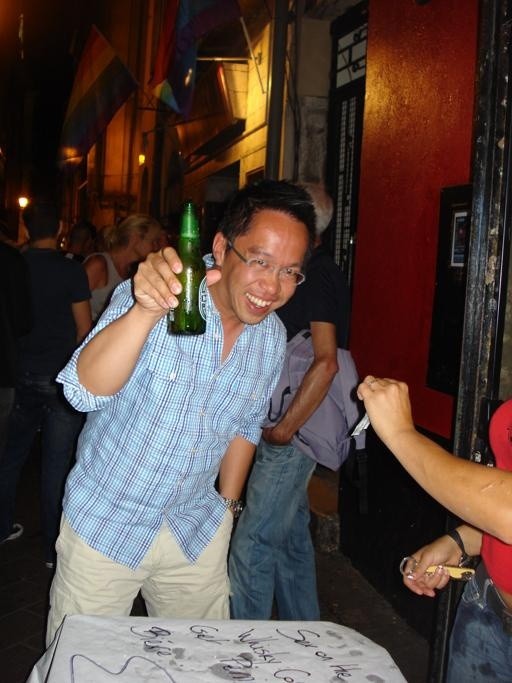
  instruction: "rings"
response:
[367,377,379,386]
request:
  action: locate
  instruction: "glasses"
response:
[225,238,306,287]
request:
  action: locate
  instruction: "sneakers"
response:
[0,522,24,545]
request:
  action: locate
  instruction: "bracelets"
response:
[447,524,473,566]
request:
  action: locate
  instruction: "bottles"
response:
[166,197,207,340]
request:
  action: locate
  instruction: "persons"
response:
[357,374,512,683]
[229,181,337,619]
[44,178,310,647]
[2,173,172,571]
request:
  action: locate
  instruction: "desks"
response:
[26,609,407,683]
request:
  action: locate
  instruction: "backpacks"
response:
[261,327,368,472]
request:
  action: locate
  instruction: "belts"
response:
[473,559,512,636]
